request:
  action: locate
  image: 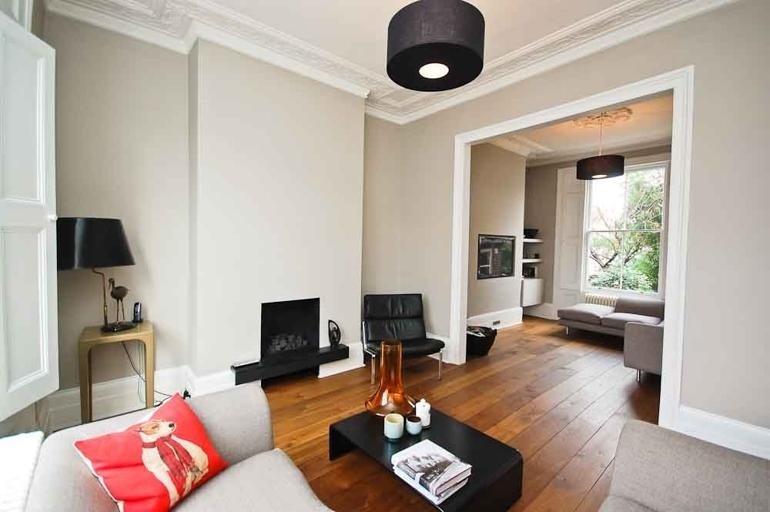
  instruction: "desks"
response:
[78,322,156,424]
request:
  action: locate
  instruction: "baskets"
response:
[467,326,497,357]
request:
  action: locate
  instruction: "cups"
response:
[416,398,432,428]
[384,413,404,440]
[406,415,423,436]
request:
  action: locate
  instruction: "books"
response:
[394,464,469,507]
[391,439,472,496]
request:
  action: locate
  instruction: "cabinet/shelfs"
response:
[521,238,544,309]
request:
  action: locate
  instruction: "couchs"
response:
[557,294,664,384]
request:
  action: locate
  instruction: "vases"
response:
[365,340,417,420]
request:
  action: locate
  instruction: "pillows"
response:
[72,393,232,512]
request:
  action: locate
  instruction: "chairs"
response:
[23,380,335,512]
[361,292,447,386]
[594,418,770,512]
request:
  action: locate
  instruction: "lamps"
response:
[386,1,484,94]
[56,215,137,333]
[575,111,625,181]
